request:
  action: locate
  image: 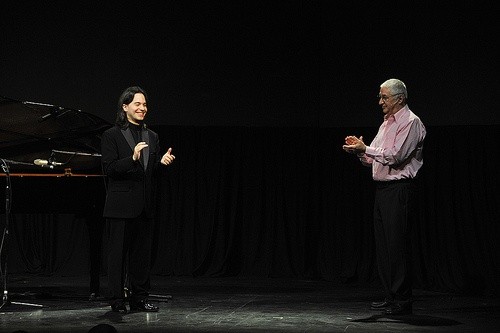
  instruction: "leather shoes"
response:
[386,304,411,314]
[130,303,159,312]
[371,298,411,314]
[111,303,127,314]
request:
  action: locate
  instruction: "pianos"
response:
[0,95,113,298]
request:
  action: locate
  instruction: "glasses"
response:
[377,93,403,100]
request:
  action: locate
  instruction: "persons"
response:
[343,79,427,313]
[103,86,176,312]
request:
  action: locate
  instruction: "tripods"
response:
[0,158,48,312]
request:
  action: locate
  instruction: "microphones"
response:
[34,159,64,167]
